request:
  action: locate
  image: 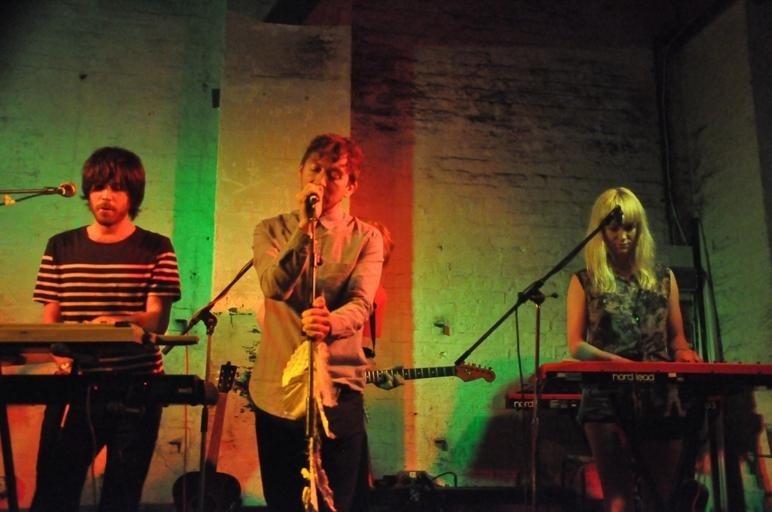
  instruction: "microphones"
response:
[307,193,319,204]
[57,181,76,197]
[610,206,625,231]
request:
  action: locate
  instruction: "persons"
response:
[567,187,702,512]
[248,135,384,512]
[29,147,181,512]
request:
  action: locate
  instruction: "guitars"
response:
[365,362,495,384]
[172,362,243,510]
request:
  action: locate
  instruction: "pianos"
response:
[506,360,771,414]
[0,322,219,405]
